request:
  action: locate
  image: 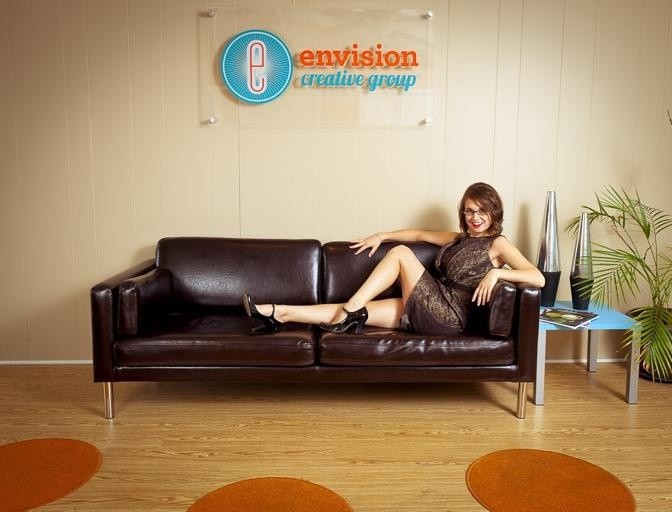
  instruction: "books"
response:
[539,306,601,330]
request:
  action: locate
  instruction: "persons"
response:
[244,182,546,335]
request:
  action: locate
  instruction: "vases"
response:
[536,190,561,307]
[570,212,594,310]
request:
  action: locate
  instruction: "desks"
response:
[533,301,644,404]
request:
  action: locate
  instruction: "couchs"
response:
[90,237,542,419]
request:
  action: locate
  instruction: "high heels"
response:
[319,306,368,334]
[243,293,284,334]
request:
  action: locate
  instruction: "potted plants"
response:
[563,186,672,383]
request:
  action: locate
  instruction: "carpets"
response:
[465,448,636,512]
[185,477,353,512]
[0,438,102,512]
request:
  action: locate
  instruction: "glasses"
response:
[462,209,487,216]
